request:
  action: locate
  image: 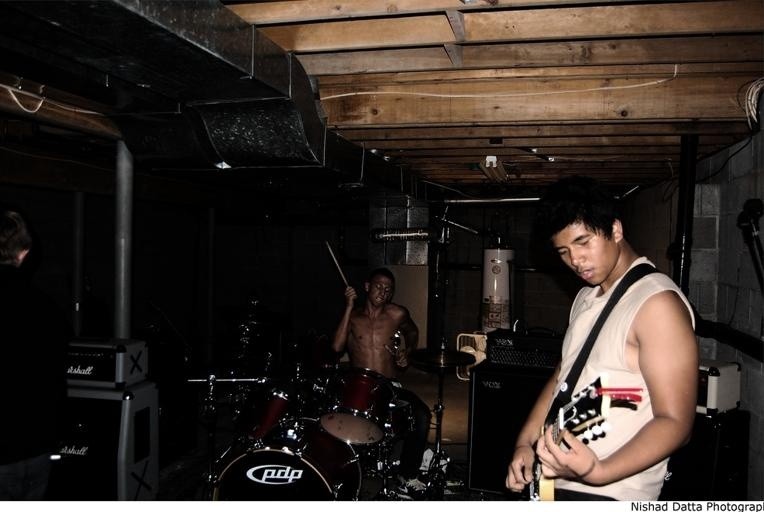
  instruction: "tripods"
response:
[413,338,467,501]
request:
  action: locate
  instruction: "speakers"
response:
[67,337,149,390]
[668,409,750,501]
[58,385,159,501]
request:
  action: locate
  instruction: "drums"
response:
[313,367,392,445]
[211,418,360,500]
[231,385,291,452]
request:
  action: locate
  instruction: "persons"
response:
[503,176,701,500]
[330,268,419,379]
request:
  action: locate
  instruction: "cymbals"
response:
[402,348,475,374]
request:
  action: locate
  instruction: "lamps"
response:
[478,153,512,185]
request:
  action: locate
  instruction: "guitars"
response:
[521,387,609,501]
